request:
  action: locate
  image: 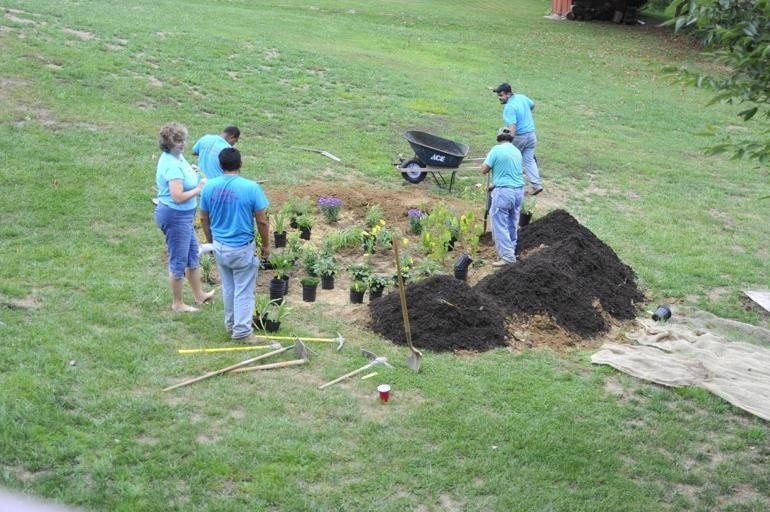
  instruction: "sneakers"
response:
[238,336,265,344]
[492,259,509,267]
[528,185,543,195]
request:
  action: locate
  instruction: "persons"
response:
[198,146,271,343]
[154,120,216,313]
[191,125,240,255]
[480,126,524,267]
[492,83,543,196]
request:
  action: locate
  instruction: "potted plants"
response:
[250,195,481,333]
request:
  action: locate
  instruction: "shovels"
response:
[392,237,423,374]
[478,171,495,247]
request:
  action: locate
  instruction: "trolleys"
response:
[396,130,488,183]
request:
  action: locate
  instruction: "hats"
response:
[493,83,511,92]
[497,127,512,136]
